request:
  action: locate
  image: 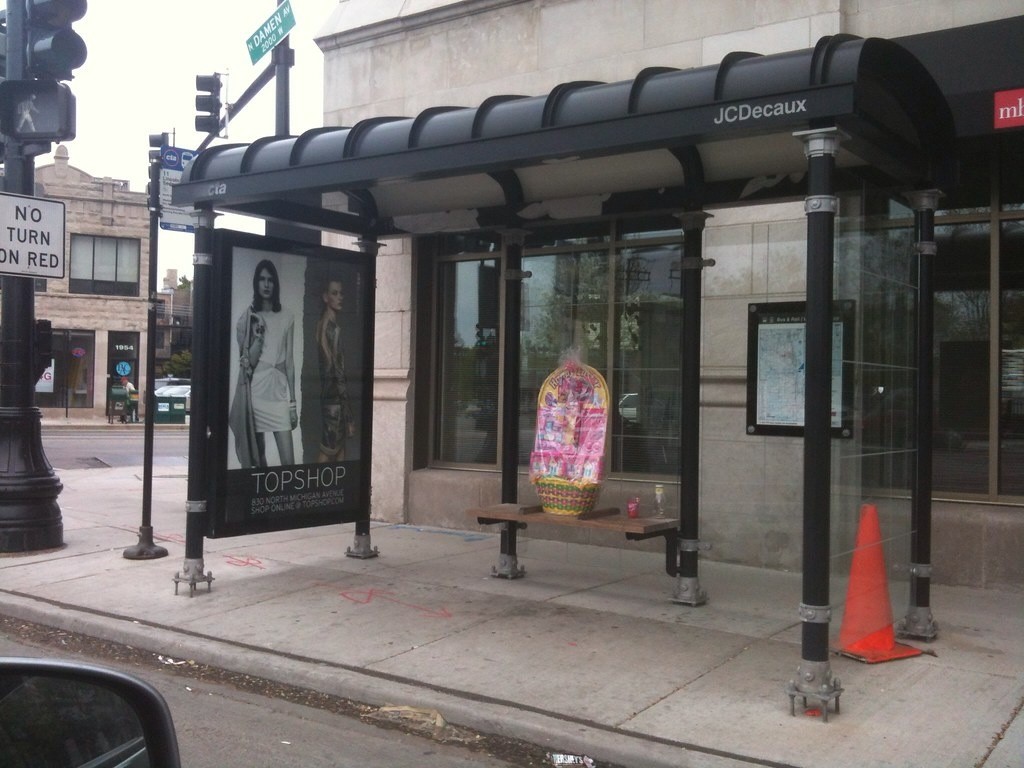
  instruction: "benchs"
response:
[465,502,681,579]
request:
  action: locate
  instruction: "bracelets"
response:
[254,325,265,343]
[289,399,296,412]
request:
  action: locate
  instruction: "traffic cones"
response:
[829,503,922,664]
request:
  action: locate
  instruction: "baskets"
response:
[530,477,604,516]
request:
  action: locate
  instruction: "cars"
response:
[154,377,192,391]
[616,393,638,422]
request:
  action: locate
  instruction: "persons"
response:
[314,267,357,462]
[120,377,136,398]
[227,259,298,468]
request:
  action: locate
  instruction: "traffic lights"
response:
[196,72,228,139]
[0,0,87,141]
[147,133,169,211]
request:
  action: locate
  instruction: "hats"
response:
[120,377,127,382]
